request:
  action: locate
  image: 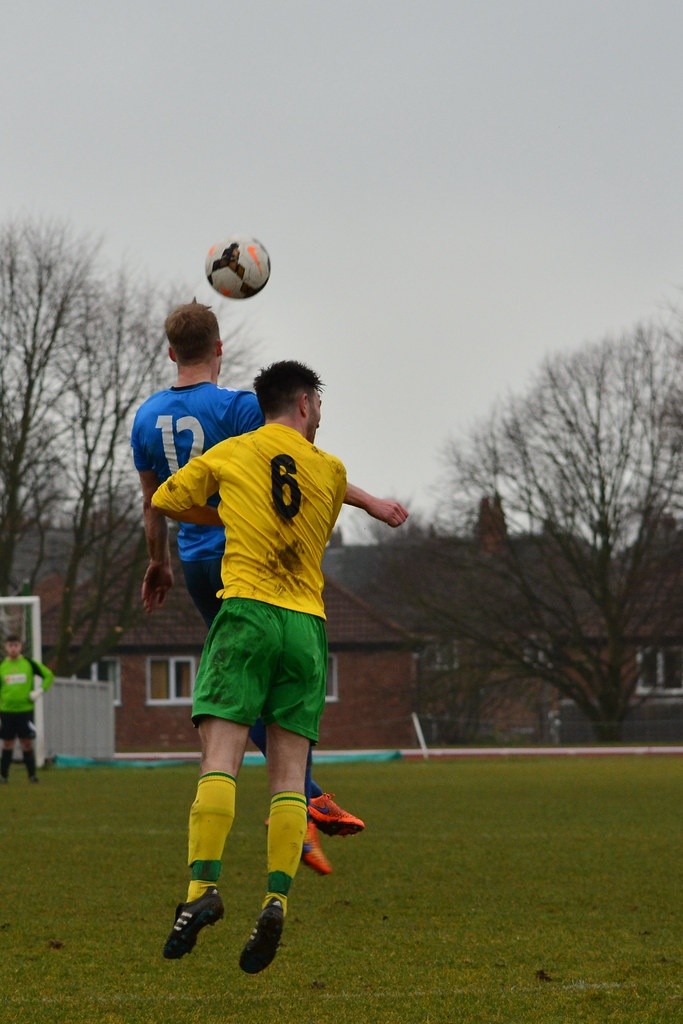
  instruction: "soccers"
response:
[204,230,273,300]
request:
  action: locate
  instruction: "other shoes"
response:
[0,774,7,784]
[29,776,38,783]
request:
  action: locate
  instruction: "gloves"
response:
[30,687,44,701]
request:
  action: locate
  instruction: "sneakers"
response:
[265,816,333,874]
[240,892,288,974]
[163,884,225,959]
[308,792,365,837]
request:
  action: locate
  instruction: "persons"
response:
[0,634,54,785]
[151,361,346,972]
[130,302,408,875]
